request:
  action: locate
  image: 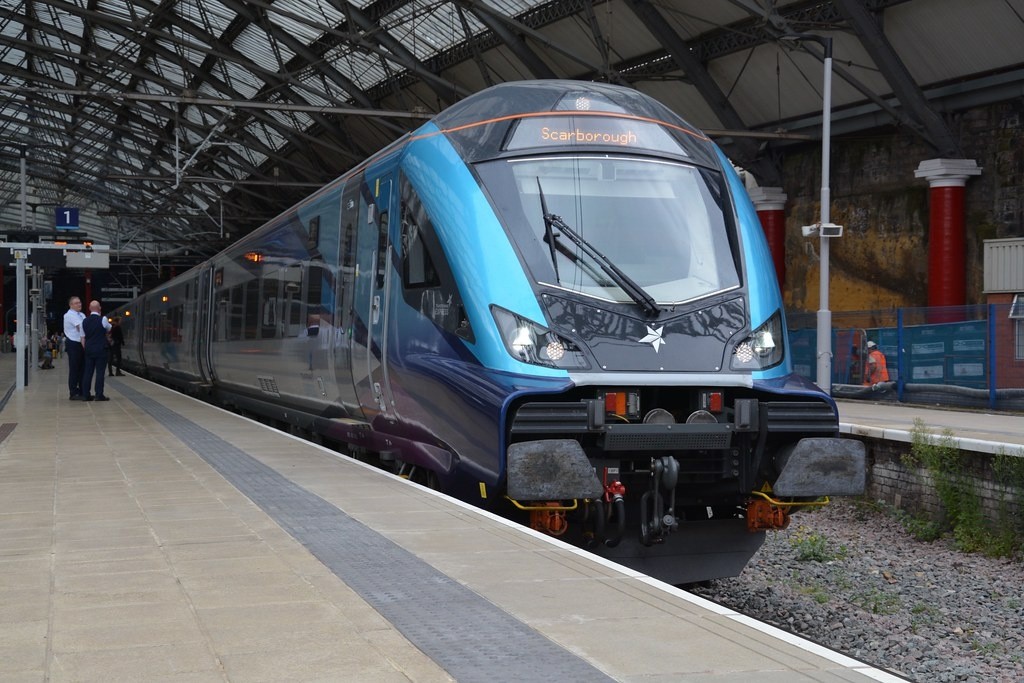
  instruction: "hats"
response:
[865,341,876,349]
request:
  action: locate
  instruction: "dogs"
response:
[37,347,55,370]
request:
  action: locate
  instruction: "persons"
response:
[863,340,890,385]
[62,297,88,401]
[80,300,112,401]
[106,316,127,377]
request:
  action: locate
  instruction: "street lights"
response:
[2,141,54,393]
[6,200,59,372]
[777,33,833,396]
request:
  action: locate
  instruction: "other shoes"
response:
[116,371,126,376]
[98,396,110,401]
[88,395,96,400]
[69,391,79,400]
[108,372,115,376]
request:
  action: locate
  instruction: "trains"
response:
[104,79,865,586]
